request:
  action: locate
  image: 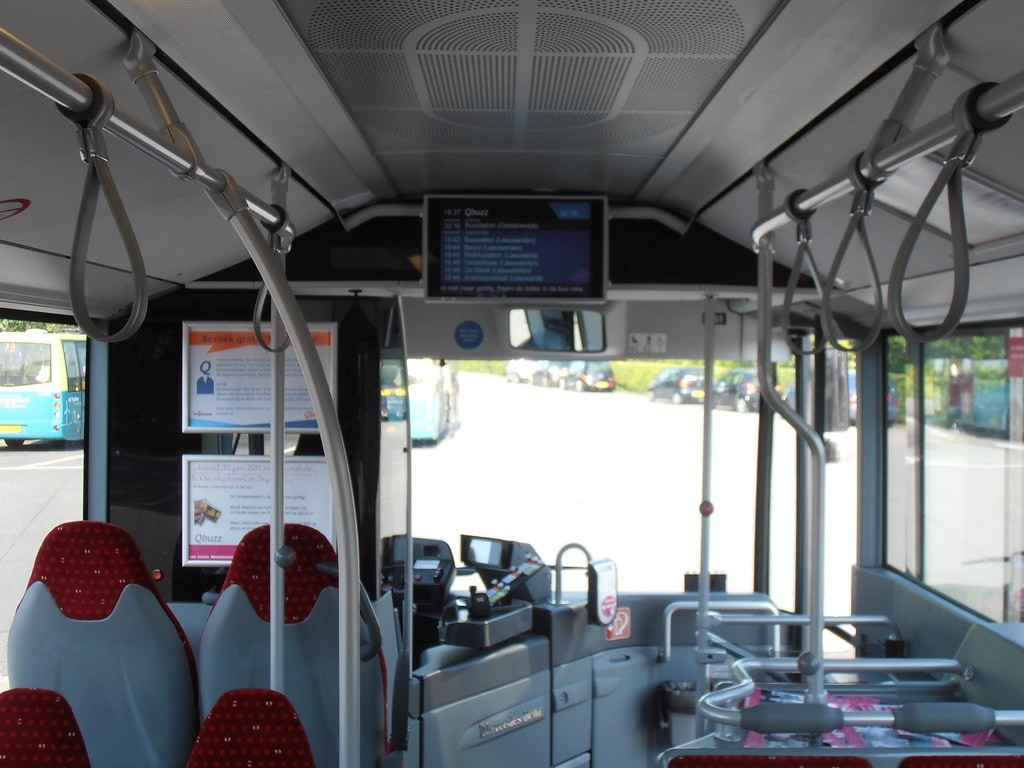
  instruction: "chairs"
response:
[0,521,390,768]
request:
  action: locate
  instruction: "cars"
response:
[707,368,781,414]
[775,369,900,432]
[504,359,616,394]
[648,365,714,405]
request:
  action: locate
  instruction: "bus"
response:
[0,327,87,449]
[382,357,461,445]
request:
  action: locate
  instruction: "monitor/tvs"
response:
[423,195,609,308]
